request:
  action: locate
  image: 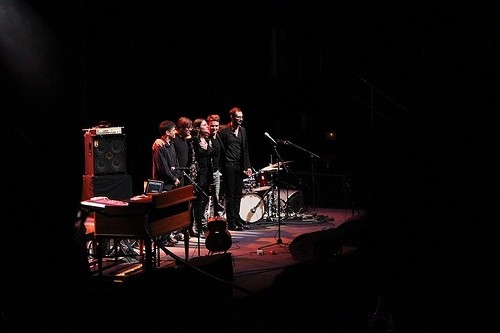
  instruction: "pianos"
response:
[80,185,198,276]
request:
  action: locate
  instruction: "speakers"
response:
[84,133,127,175]
[269,188,306,215]
[82,174,132,217]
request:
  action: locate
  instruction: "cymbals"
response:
[267,161,291,168]
[254,185,271,192]
[261,168,283,172]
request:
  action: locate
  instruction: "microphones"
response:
[265,131,277,144]
[170,166,193,171]
[277,139,288,145]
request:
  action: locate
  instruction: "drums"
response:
[239,193,265,223]
[242,176,257,195]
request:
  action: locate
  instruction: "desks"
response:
[94,185,197,271]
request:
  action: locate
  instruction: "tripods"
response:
[287,141,329,222]
[256,143,290,249]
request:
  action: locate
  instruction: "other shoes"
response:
[166,236,179,243]
[229,225,244,231]
[239,223,251,229]
[160,240,177,247]
[190,231,200,237]
[175,234,186,241]
[200,232,207,238]
[181,229,191,239]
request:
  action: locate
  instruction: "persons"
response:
[153,108,252,246]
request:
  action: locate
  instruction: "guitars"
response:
[201,169,227,231]
[204,182,233,253]
